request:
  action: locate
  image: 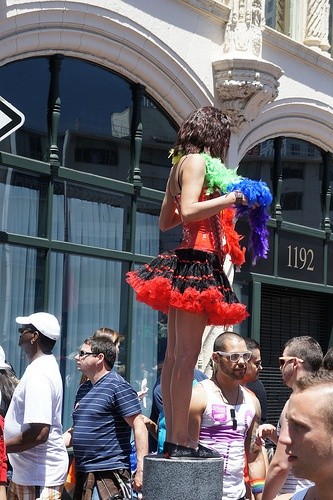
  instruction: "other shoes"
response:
[162,442,177,458]
[170,444,223,460]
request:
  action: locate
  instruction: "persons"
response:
[159,105,260,460]
[188,331,266,500]
[278,367,333,500]
[0,343,19,500]
[153,368,210,456]
[240,339,267,426]
[62,335,149,500]
[3,312,70,500]
[79,328,158,433]
[254,335,324,500]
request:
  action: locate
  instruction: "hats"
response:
[16,312,60,340]
[0,346,9,369]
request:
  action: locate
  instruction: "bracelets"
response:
[234,190,243,206]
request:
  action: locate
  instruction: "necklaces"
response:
[212,368,240,430]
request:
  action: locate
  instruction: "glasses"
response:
[18,328,34,333]
[216,350,252,362]
[230,409,237,430]
[79,351,98,356]
[279,357,304,365]
